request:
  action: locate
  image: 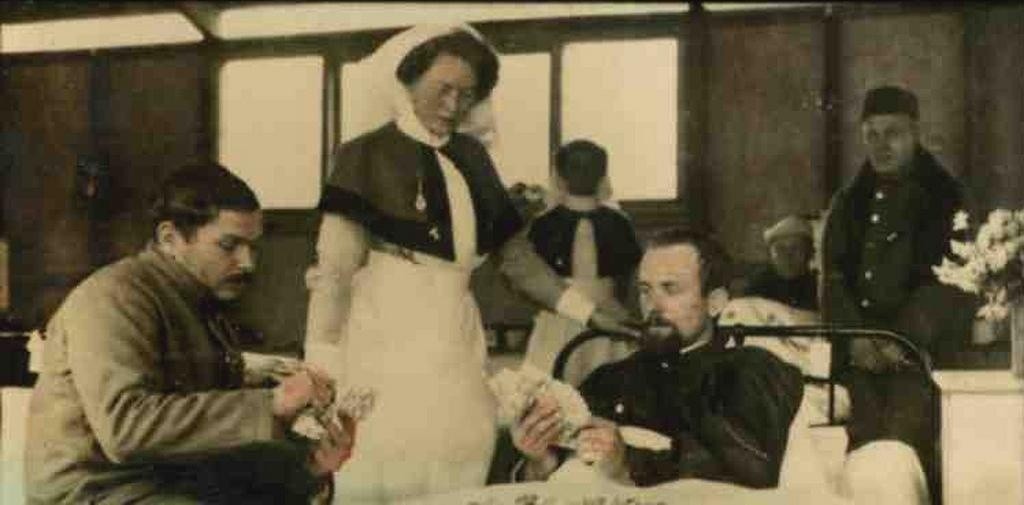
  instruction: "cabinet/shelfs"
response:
[927,366,1023,504]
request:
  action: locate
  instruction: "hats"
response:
[763,216,814,246]
[861,86,919,123]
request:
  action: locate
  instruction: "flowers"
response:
[929,203,1024,330]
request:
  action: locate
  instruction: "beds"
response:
[511,263,940,505]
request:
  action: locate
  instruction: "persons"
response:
[22,157,358,505]
[485,226,805,492]
[518,138,642,377]
[299,12,645,505]
[729,215,819,312]
[819,87,982,505]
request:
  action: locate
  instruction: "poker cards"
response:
[467,493,665,505]
[488,361,592,450]
[291,381,377,439]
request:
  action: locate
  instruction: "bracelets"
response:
[554,285,598,327]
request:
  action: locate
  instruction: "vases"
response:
[1008,302,1023,379]
